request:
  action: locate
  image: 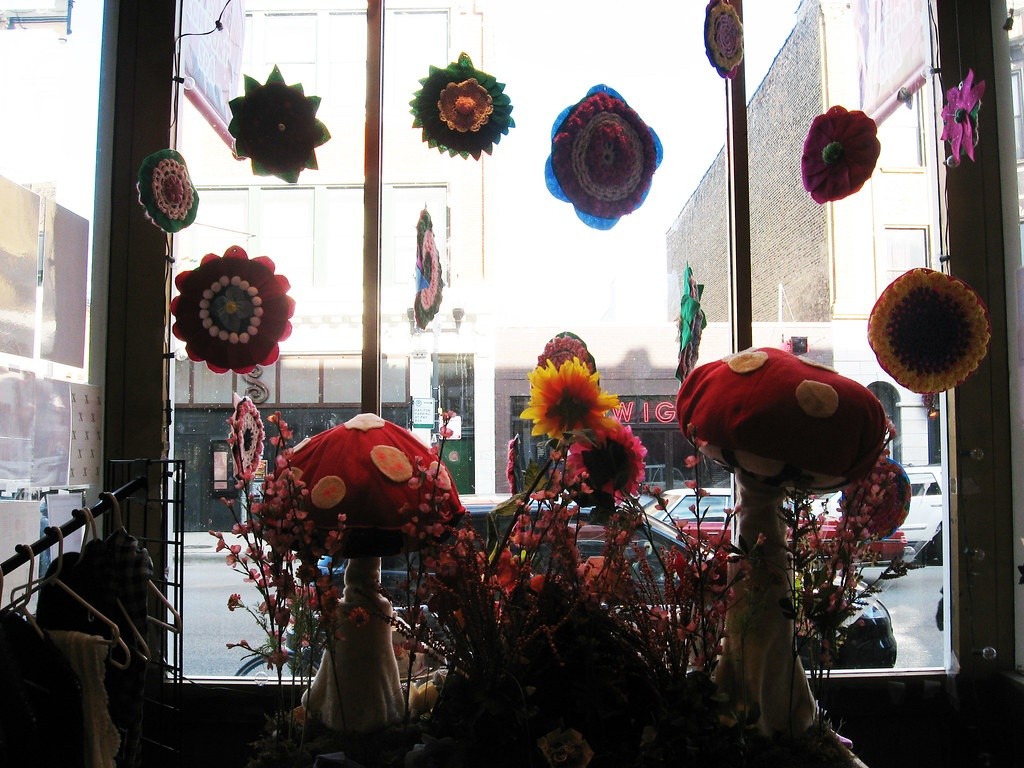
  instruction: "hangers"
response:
[0,491,182,699]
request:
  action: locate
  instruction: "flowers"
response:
[209,358,907,768]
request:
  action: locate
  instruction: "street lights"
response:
[408,307,464,450]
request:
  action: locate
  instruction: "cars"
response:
[641,487,906,568]
[829,465,944,544]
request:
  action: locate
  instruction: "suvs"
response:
[284,498,899,675]
